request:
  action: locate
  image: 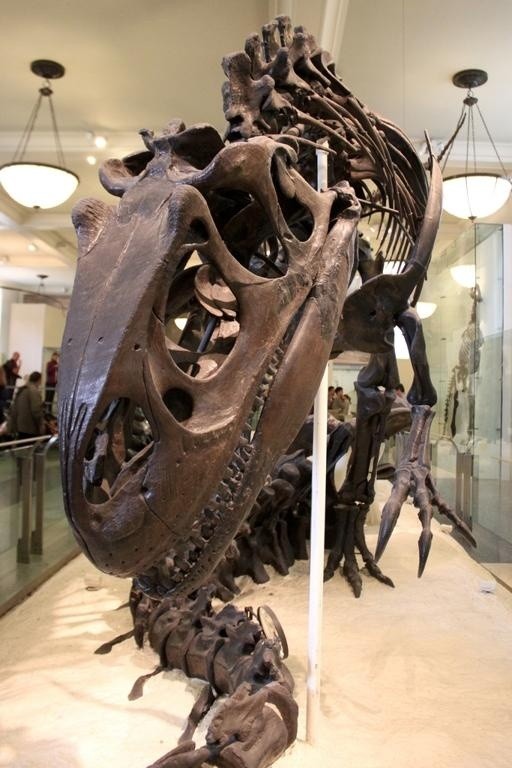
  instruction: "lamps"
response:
[1,57,83,213]
[417,67,511,227]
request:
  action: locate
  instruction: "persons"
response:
[6,371,44,504]
[0,351,23,404]
[42,351,60,418]
[326,385,352,425]
[394,383,407,402]
